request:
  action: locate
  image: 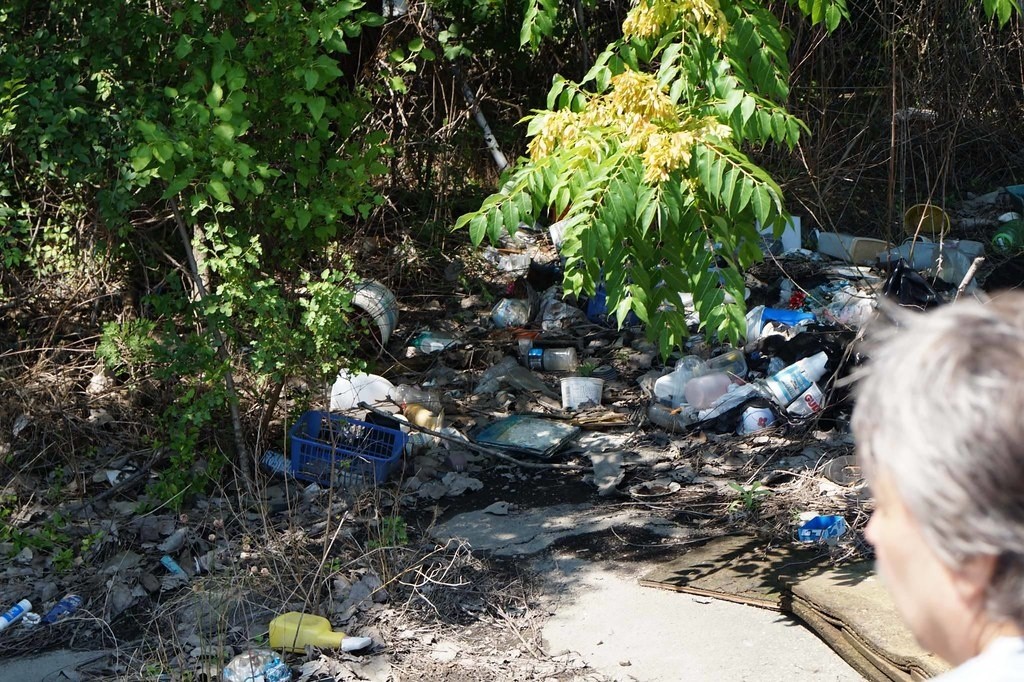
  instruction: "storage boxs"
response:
[291,410,409,487]
[697,349,748,379]
[818,232,897,265]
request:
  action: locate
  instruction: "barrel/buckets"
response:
[349,281,400,362]
[560,377,605,410]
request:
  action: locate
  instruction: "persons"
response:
[855,291,1024,682]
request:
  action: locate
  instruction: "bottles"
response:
[0,598,32,631]
[520,346,577,369]
[648,344,826,431]
[473,355,517,394]
[392,385,442,454]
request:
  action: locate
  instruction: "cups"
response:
[787,382,827,420]
